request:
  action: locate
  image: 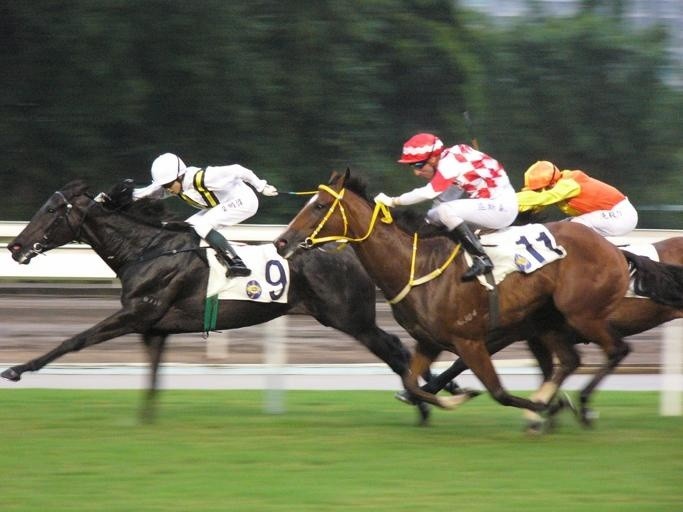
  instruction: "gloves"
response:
[373,191,399,207]
[93,192,106,203]
[261,184,279,197]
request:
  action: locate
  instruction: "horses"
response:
[273,167,683,428]
[0,181,485,425]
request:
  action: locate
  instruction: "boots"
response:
[218,247,252,278]
[449,221,494,281]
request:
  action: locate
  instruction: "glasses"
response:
[409,161,428,169]
[160,180,176,188]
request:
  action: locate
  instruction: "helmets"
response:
[150,152,187,188]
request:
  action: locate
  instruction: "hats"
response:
[521,160,563,191]
[397,132,445,164]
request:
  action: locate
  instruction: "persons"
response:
[513,160,639,238]
[371,132,522,282]
[92,151,280,278]
